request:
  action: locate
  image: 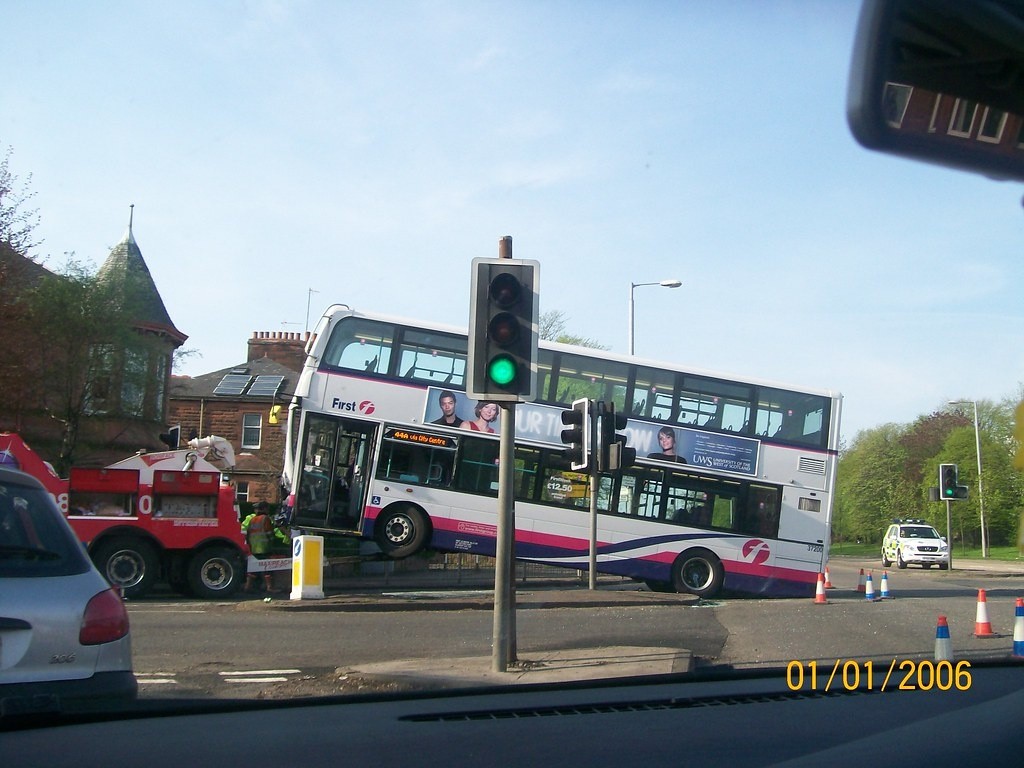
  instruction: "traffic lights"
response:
[560,397,585,471]
[940,464,958,500]
[466,258,542,403]
[605,402,636,473]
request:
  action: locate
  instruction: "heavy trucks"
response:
[0,428,406,600]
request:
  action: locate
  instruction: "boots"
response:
[244,573,254,591]
[264,574,274,592]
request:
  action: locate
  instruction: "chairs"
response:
[363,355,787,441]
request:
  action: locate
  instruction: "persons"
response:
[239,501,313,591]
[431,390,463,428]
[646,427,688,464]
[459,401,499,434]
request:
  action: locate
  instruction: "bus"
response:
[271,304,843,603]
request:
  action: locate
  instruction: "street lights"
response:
[947,400,987,559]
[630,279,683,355]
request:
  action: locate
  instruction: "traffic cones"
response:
[931,615,959,664]
[855,568,866,593]
[879,571,896,600]
[970,589,1001,638]
[822,566,832,589]
[863,570,883,602]
[813,572,830,605]
[1006,597,1024,658]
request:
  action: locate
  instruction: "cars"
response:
[0,468,141,703]
[881,518,949,570]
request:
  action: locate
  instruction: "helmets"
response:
[251,503,258,508]
